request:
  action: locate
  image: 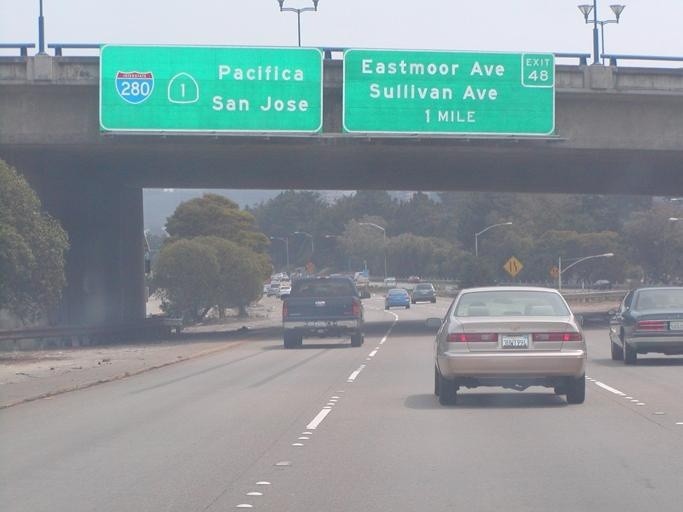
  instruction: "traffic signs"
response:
[97,44,324,136]
[341,49,556,139]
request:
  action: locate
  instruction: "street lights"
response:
[474,222,512,259]
[293,231,313,253]
[577,2,625,64]
[325,235,351,271]
[557,252,614,291]
[277,0,320,45]
[666,217,682,222]
[671,197,682,202]
[269,236,289,268]
[359,222,387,277]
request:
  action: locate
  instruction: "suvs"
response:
[411,283,437,304]
[382,288,409,310]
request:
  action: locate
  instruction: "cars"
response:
[425,283,589,409]
[262,267,373,349]
[604,285,682,365]
[408,274,423,283]
[384,277,396,287]
[624,278,641,290]
[592,278,612,290]
